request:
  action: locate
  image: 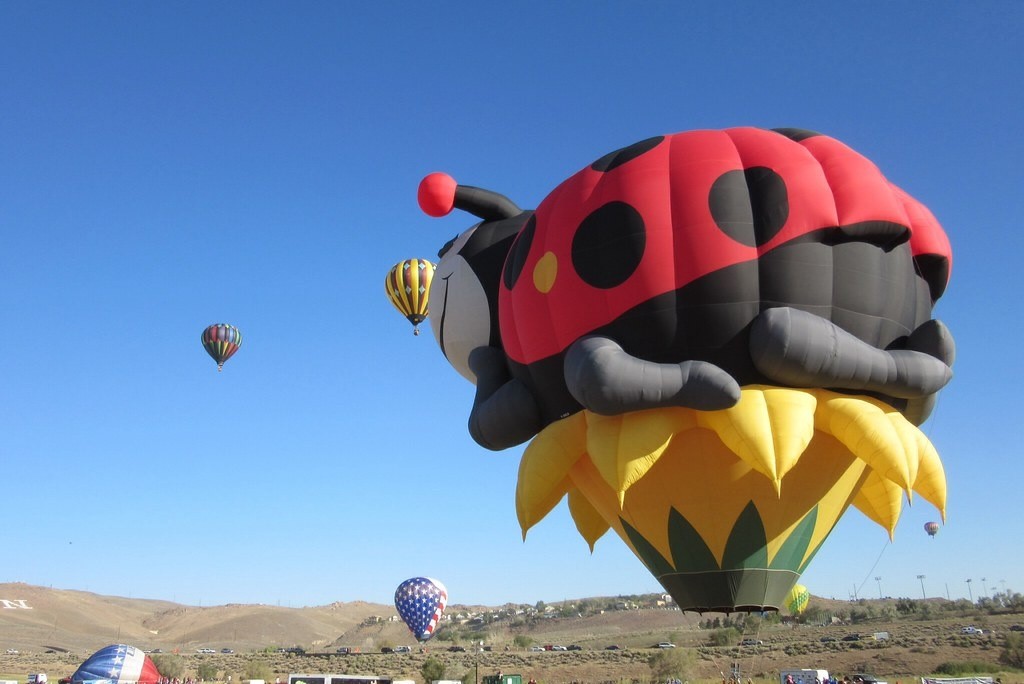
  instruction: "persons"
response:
[787,673,797,684]
[722,676,726,684]
[275,674,280,684]
[528,677,537,684]
[728,675,741,684]
[666,677,688,684]
[228,675,231,684]
[993,678,1001,684]
[160,675,200,684]
[814,674,851,684]
[797,677,803,684]
[747,676,752,684]
[498,670,503,679]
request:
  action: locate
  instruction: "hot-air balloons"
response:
[70,644,169,684]
[384,256,438,336]
[924,521,939,540]
[201,323,244,371]
[394,576,448,652]
[787,583,810,620]
[416,124,955,684]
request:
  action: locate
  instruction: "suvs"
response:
[961,626,983,635]
[658,641,676,648]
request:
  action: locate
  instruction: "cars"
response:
[196,647,305,654]
[394,645,411,652]
[605,645,619,651]
[448,643,583,652]
[5,649,18,655]
[337,647,348,653]
[381,646,393,653]
[843,673,888,684]
[58,676,71,684]
[842,636,860,641]
[821,636,836,642]
[741,638,763,645]
[1009,625,1024,631]
